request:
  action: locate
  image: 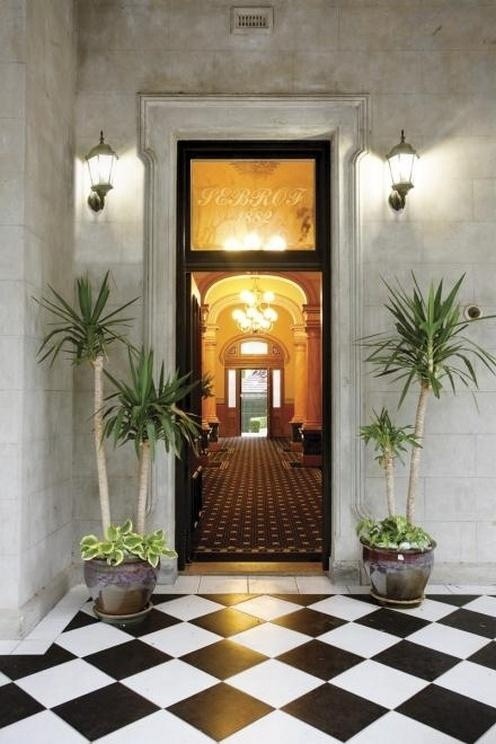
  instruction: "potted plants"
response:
[80,523,179,611]
[357,515,436,600]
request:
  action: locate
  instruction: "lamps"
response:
[386,128,421,212]
[79,129,120,214]
[232,287,278,337]
